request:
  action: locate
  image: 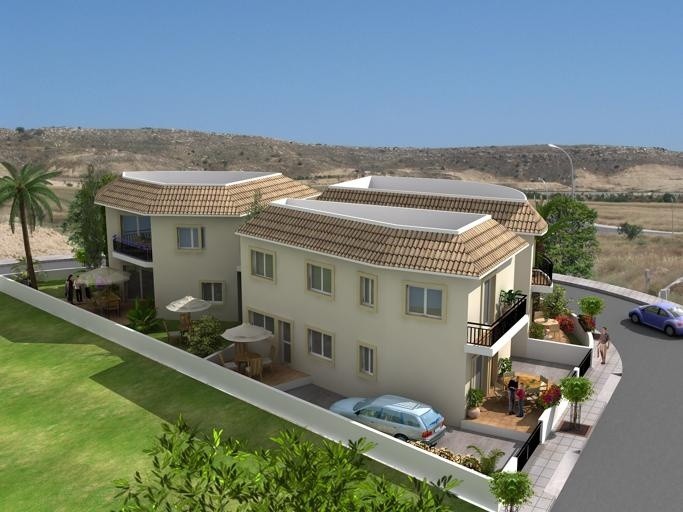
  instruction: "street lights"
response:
[529,175,547,200]
[547,143,574,200]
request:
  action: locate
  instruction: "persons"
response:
[514,383,525,418]
[65,274,91,305]
[595,327,609,364]
[507,376,518,416]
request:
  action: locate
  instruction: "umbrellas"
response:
[165,296,211,328]
[220,321,272,374]
[79,265,132,298]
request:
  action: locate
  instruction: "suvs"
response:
[328,394,445,450]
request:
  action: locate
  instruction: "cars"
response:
[628,302,683,337]
